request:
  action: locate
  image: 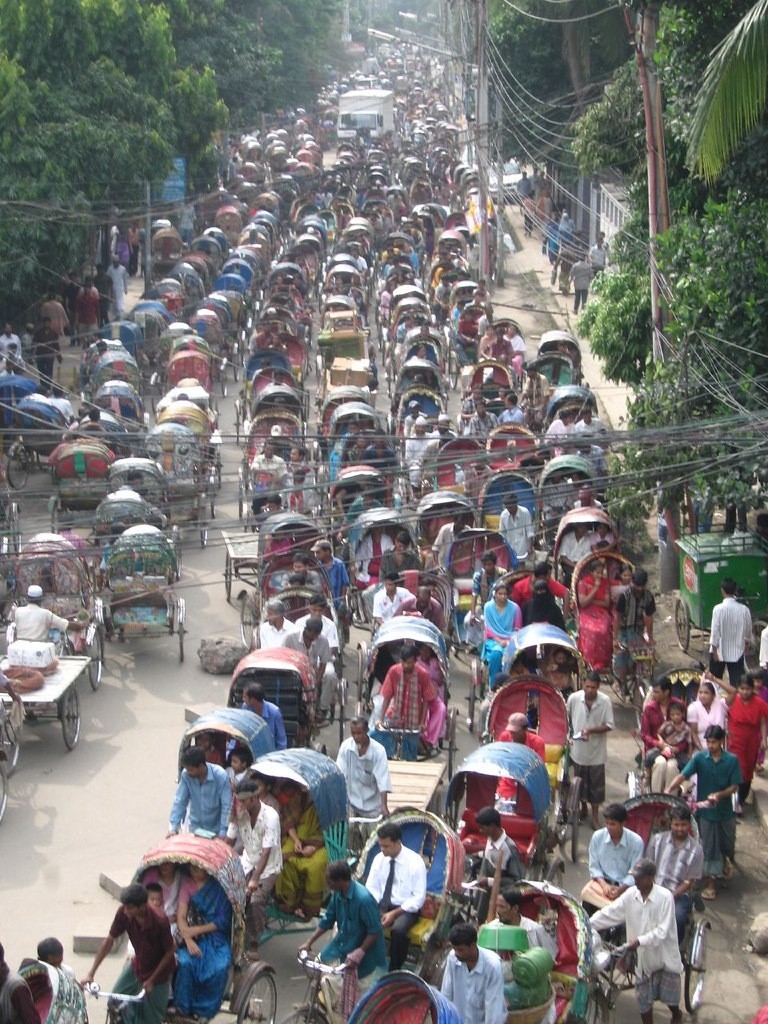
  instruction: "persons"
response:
[140,862,232,1020]
[639,668,768,817]
[664,725,744,900]
[497,711,545,800]
[37,937,76,981]
[490,885,559,961]
[0,942,41,1024]
[166,747,232,841]
[646,805,704,943]
[197,732,248,786]
[249,772,329,922]
[0,168,768,761]
[365,822,427,972]
[232,783,283,962]
[437,923,508,1024]
[229,35,459,168]
[334,717,394,835]
[296,860,390,1008]
[582,798,644,941]
[80,884,177,1024]
[565,670,615,831]
[473,807,524,889]
[589,858,684,1024]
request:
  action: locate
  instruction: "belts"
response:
[605,878,619,885]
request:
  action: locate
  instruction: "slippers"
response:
[722,864,732,880]
[702,887,716,900]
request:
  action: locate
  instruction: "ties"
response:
[379,860,395,915]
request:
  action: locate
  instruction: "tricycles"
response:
[1,48,768,1024]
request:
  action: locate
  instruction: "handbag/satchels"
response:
[581,879,618,909]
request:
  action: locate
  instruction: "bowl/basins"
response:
[478,926,529,950]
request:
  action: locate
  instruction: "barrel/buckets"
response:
[512,946,553,985]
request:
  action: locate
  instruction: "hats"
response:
[396,531,411,545]
[310,540,331,552]
[438,414,449,422]
[505,712,528,733]
[415,416,426,425]
[267,601,284,613]
[271,425,282,437]
[408,400,421,409]
[631,569,648,584]
[625,857,656,877]
[28,585,43,598]
